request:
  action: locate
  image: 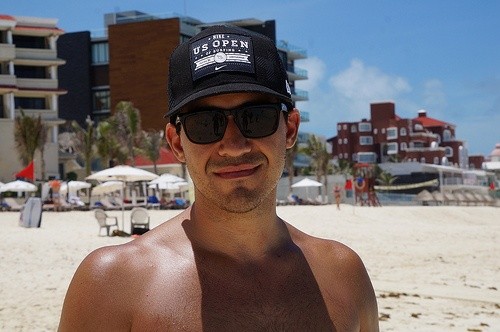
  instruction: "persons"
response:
[49,176,61,212]
[57,25,379,332]
[369,185,377,206]
[333,184,341,209]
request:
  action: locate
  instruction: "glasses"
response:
[174,100,289,143]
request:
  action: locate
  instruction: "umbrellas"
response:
[291,178,323,200]
[59,180,91,195]
[149,173,189,200]
[85,165,160,232]
[0,180,38,198]
[91,181,125,206]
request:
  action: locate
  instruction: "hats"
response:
[162,24,292,117]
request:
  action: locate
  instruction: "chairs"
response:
[94,209,120,236]
[130,208,150,234]
[100,195,161,210]
[5,197,85,212]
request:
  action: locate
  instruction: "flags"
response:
[489,181,495,191]
[16,161,34,181]
[342,178,353,190]
